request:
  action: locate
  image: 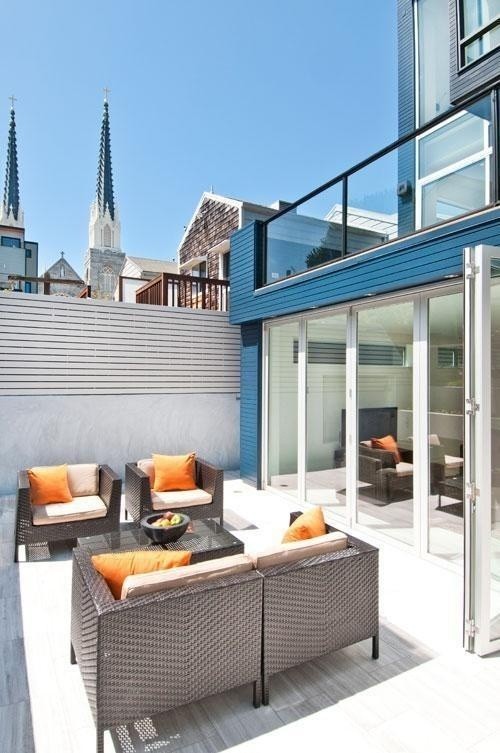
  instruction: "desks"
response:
[434,473,463,513]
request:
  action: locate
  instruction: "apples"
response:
[150,511,183,526]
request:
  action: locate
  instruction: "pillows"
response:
[370,435,400,465]
[120,553,252,601]
[137,458,155,488]
[255,531,348,569]
[280,505,326,544]
[26,464,72,504]
[151,451,196,492]
[67,464,99,497]
[91,550,192,600]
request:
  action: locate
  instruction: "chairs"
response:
[69,545,261,749]
[408,434,463,480]
[124,456,224,529]
[258,510,379,706]
[15,464,122,562]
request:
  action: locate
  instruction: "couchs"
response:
[343,444,412,497]
